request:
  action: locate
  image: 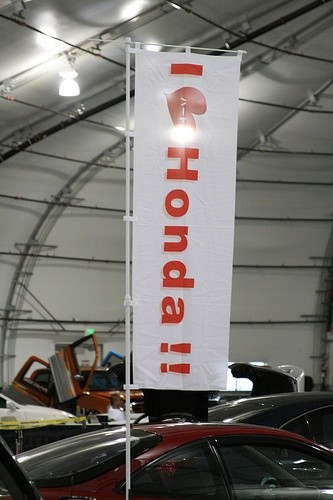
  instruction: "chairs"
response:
[131,467,164,495]
[172,464,207,496]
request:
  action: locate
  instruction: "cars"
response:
[0,420,333,500]
[168,390,333,449]
[0,393,78,428]
[100,347,226,411]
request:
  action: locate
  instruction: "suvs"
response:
[4,333,145,424]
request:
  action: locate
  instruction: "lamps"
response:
[58,69,81,98]
